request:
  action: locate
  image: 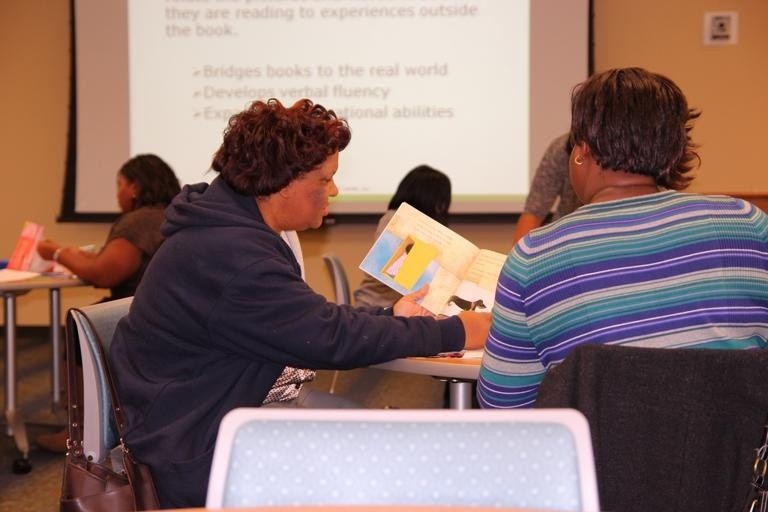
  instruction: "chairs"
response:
[70,291,135,475]
[280,229,306,282]
[204,406,601,512]
[323,255,353,393]
[534,341,768,511]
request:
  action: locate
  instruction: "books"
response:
[356,202,506,319]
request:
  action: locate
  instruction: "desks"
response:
[1,270,91,474]
[367,348,487,410]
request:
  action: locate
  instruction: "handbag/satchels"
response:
[60,451,161,512]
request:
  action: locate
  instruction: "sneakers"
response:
[35,430,83,454]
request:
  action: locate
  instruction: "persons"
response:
[514,129,583,252]
[356,165,454,316]
[473,65,767,408]
[35,154,182,454]
[106,98,498,510]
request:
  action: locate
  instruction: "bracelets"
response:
[51,246,65,261]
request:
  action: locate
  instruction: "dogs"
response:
[445,295,486,316]
